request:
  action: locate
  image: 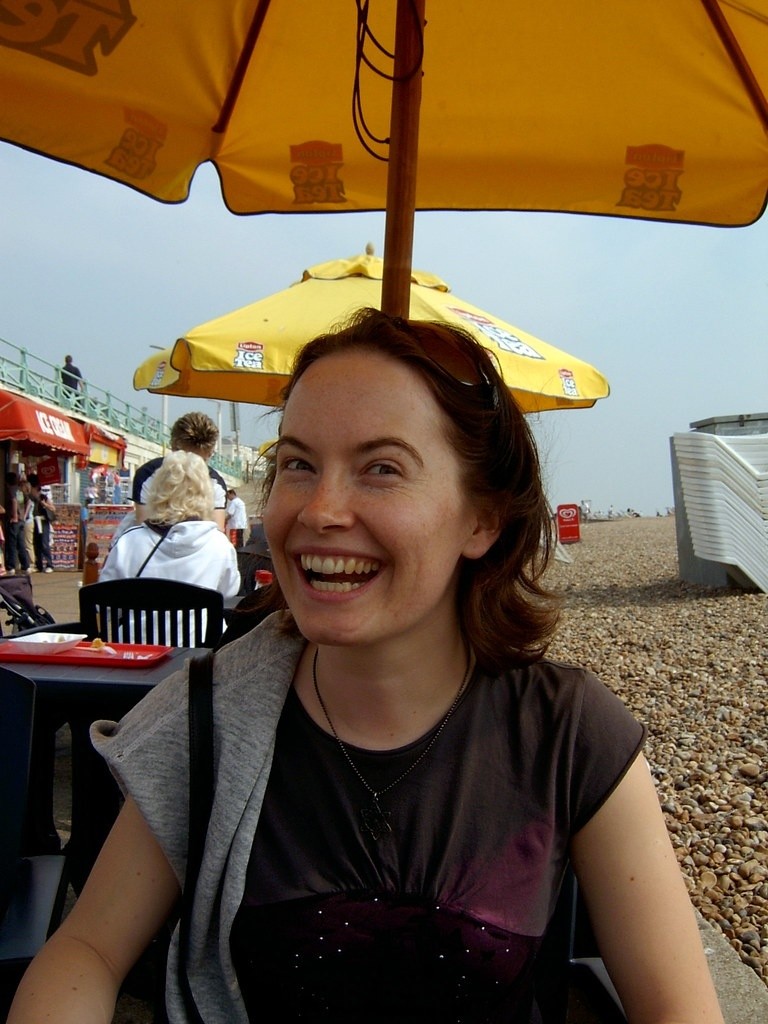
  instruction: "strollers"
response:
[0,571,55,635]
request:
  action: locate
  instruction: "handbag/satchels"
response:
[45,508,56,521]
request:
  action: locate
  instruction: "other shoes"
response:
[6,569,16,574]
[44,567,55,573]
[26,568,31,573]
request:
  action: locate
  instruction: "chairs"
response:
[78,577,224,650]
[0,665,37,858]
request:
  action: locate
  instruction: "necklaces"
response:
[313,640,470,841]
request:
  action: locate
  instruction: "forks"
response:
[123,651,134,659]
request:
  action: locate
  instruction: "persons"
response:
[0,472,56,575]
[6,318,727,1024]
[224,489,245,546]
[61,354,84,412]
[97,413,241,648]
[81,498,92,546]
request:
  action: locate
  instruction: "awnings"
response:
[0,389,90,457]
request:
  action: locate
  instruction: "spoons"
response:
[74,646,117,655]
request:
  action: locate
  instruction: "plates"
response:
[9,632,88,654]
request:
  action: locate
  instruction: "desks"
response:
[0,645,213,854]
[0,855,69,972]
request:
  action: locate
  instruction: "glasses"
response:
[404,320,500,408]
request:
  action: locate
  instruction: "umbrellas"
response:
[132,242,611,413]
[0,0,768,319]
